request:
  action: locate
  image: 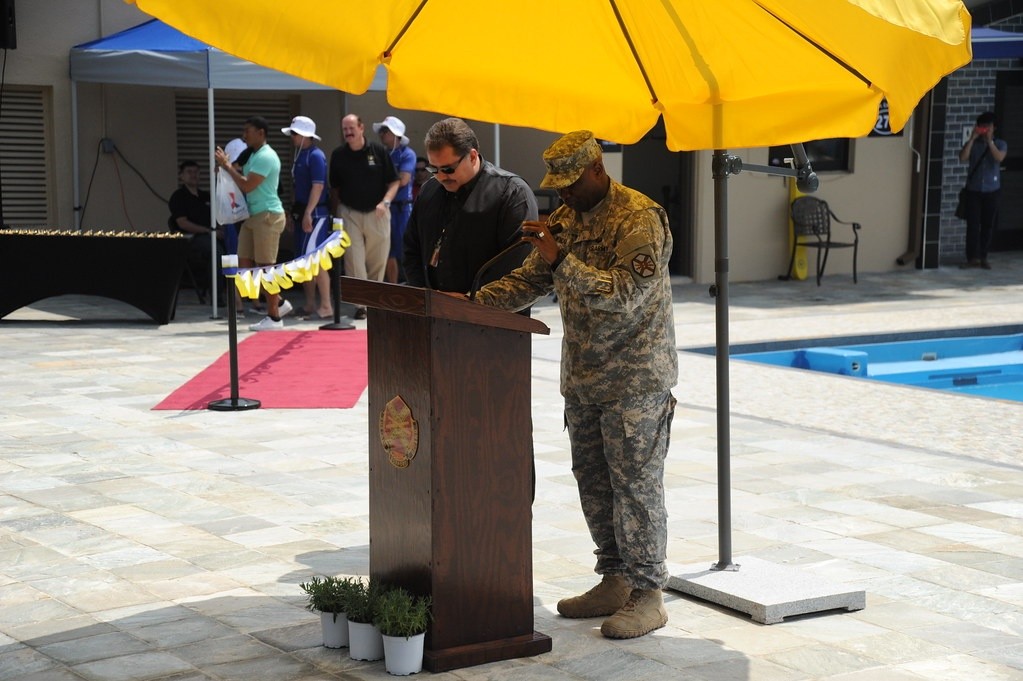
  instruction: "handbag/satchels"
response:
[954,188,968,220]
[290,201,321,222]
[216,166,249,225]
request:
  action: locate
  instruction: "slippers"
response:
[288,307,334,321]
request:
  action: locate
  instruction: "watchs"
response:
[381,199,391,208]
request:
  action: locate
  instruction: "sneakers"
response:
[249,316,283,331]
[557,573,633,617]
[278,299,293,318]
[601,589,668,639]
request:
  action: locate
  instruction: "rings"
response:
[537,232,545,240]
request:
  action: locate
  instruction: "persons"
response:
[437,130,679,640]
[955,110,1007,269]
[166,114,429,332]
[396,117,538,318]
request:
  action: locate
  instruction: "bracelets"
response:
[465,291,472,298]
[989,140,995,146]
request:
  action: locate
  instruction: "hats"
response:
[372,116,409,146]
[540,130,602,190]
[224,138,248,164]
[281,116,321,141]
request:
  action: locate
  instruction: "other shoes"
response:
[355,308,367,319]
[249,307,268,315]
[211,300,227,307]
[968,257,991,269]
[236,310,245,319]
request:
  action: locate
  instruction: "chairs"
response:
[777,197,860,286]
[168,216,208,306]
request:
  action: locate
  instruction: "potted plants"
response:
[372,588,438,676]
[299,572,368,648]
[338,575,392,661]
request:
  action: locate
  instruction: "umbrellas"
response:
[129,0,982,571]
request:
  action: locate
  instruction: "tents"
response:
[70,19,502,232]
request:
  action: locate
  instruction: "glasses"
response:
[415,168,425,172]
[425,156,464,175]
[379,127,389,135]
[291,130,297,136]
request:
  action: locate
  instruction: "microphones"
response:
[469,223,563,302]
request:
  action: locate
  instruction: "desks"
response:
[0,234,195,324]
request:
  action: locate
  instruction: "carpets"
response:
[152,329,368,409]
